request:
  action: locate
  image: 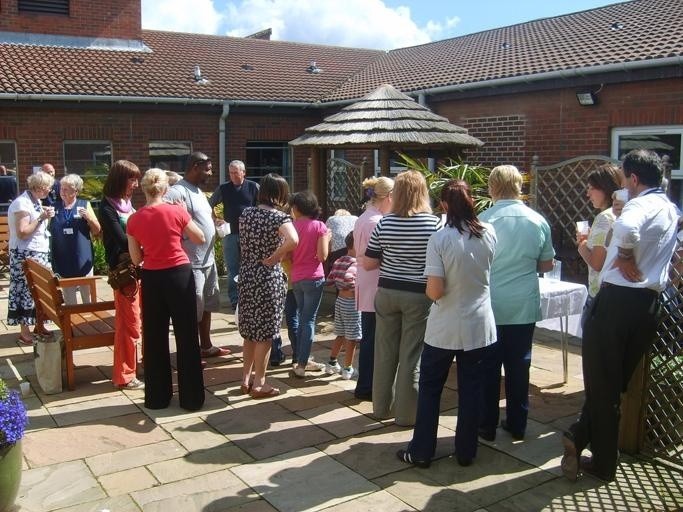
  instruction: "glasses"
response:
[195,158,214,167]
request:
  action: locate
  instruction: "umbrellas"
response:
[287,84,485,177]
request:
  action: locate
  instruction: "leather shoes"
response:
[562,430,582,482]
[579,455,615,481]
[501,419,523,440]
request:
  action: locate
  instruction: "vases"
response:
[0,438,25,511]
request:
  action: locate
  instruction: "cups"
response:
[19,381,30,398]
[614,189,628,205]
[216,227,225,238]
[543,259,561,286]
[575,220,589,236]
[222,222,231,235]
[76,206,83,217]
[46,206,55,217]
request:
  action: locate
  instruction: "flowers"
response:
[0,378,29,447]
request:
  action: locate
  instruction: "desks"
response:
[534,274,586,382]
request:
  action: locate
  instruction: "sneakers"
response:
[114,378,144,390]
[18,335,37,346]
[201,345,232,357]
[453,449,474,465]
[33,325,55,338]
[397,449,432,469]
[293,355,356,380]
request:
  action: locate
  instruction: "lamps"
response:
[574,82,607,108]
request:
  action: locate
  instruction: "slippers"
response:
[250,384,281,399]
[241,376,256,394]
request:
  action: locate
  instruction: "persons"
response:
[396,183,495,467]
[560,153,682,481]
[97,159,146,389]
[470,164,556,443]
[126,166,206,411]
[209,159,332,399]
[7,164,101,345]
[316,170,450,427]
[161,152,233,357]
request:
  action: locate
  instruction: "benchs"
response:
[18,254,141,392]
[0,216,9,267]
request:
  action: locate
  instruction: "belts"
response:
[600,280,663,300]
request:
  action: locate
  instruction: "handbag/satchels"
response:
[33,334,62,394]
[106,254,142,298]
